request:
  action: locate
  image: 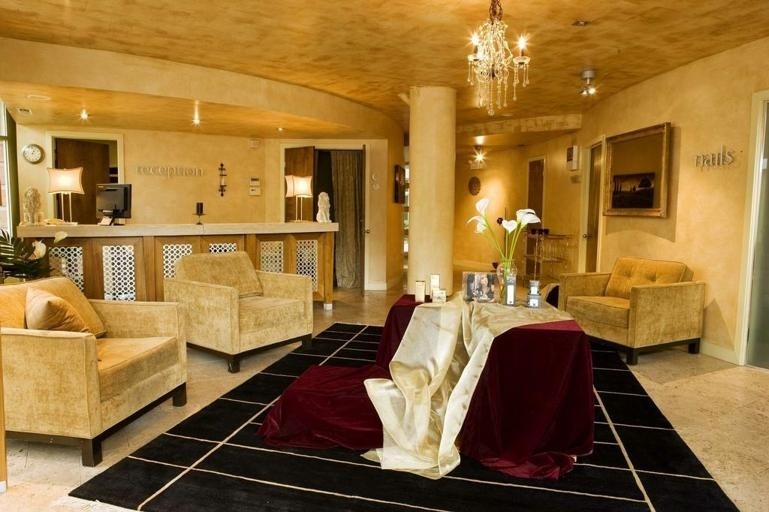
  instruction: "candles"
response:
[196,202,203,215]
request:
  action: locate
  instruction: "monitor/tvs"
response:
[96,183,131,226]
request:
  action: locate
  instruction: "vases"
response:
[495,259,518,308]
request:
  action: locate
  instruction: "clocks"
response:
[22,144,44,164]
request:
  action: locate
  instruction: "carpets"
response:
[69,321,740,511]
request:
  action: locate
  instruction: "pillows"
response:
[24,287,102,361]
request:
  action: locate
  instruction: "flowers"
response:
[466,198,542,303]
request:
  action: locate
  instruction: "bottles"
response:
[528,279,540,308]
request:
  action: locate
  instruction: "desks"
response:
[256,288,595,480]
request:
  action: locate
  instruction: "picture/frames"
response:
[394,164,406,204]
[601,122,672,217]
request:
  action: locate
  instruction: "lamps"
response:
[47,166,86,223]
[467,0,530,116]
[285,174,313,223]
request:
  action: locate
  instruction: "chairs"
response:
[0,276,188,467]
[164,251,314,373]
[558,255,706,367]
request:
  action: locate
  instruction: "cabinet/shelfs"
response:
[520,227,576,290]
